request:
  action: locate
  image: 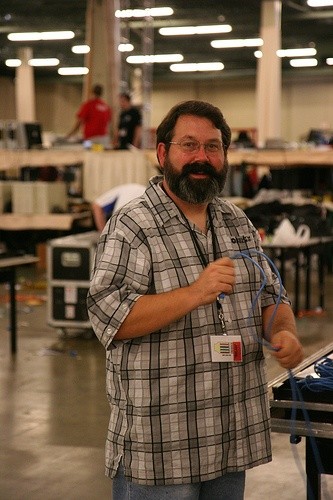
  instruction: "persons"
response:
[305,129,327,145]
[64,84,113,150]
[86,98,304,500]
[91,182,149,234]
[114,93,144,150]
[233,130,255,148]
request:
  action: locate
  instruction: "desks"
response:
[0,212,78,244]
[260,236,332,318]
[0,254,39,353]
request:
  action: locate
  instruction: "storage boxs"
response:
[269,343,333,500]
[47,231,98,331]
[10,180,69,216]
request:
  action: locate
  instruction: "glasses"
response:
[167,141,226,153]
